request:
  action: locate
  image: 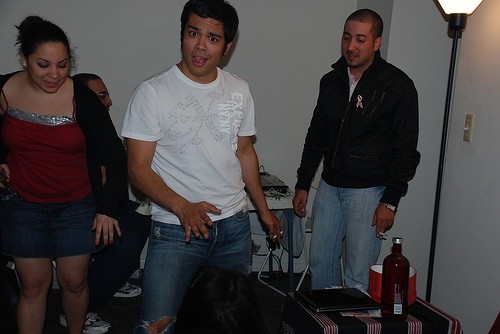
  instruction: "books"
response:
[294,288,381,313]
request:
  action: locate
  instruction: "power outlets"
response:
[462,113,475,142]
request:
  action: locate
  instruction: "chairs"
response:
[258,186,345,297]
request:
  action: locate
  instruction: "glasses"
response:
[97,91,110,98]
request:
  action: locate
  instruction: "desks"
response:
[280,288,465,334]
[247,188,294,291]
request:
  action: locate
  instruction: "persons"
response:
[59,72,143,333]
[0,14,131,332]
[290,9,424,291]
[123,0,284,334]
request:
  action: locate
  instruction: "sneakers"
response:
[60,311,112,334]
[114,282,141,298]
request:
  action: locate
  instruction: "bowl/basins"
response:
[369,264,416,306]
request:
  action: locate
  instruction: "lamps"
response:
[425,0,483,303]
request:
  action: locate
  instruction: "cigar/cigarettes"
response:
[273,231,284,239]
[379,232,387,236]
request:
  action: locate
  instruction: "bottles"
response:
[381,237,410,321]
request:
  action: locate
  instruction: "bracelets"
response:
[379,201,397,211]
